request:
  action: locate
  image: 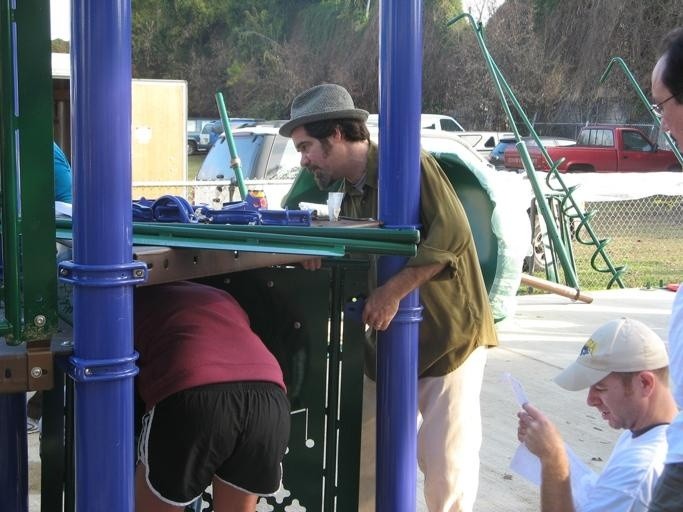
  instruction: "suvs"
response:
[489,137,577,173]
[187,119,216,156]
[197,118,265,151]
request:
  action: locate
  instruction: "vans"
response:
[366,114,465,135]
[195,123,496,210]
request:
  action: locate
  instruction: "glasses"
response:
[650,96,675,118]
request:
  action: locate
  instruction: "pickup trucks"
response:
[504,126,683,172]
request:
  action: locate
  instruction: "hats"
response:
[552,318,670,391]
[280,84,369,137]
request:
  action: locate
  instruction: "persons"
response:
[648,29,683,512]
[26,143,72,433]
[280,84,499,511]
[518,317,678,512]
[133,281,290,512]
